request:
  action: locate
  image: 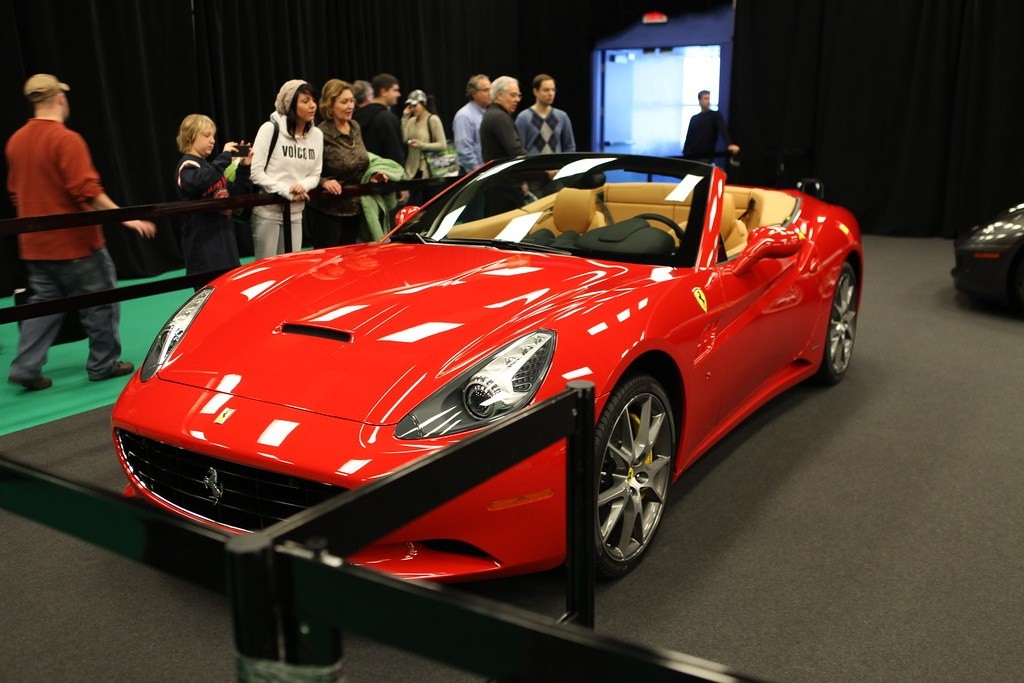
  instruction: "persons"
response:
[249,78,324,261]
[352,74,449,236]
[683,91,741,160]
[452,75,492,224]
[306,79,388,250]
[6,73,158,392]
[479,76,529,216]
[513,74,576,204]
[174,114,253,293]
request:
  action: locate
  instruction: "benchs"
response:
[596,182,761,232]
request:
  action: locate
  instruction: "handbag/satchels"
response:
[14,290,89,346]
[230,179,260,224]
[423,114,460,178]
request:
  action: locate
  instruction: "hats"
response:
[274,79,308,115]
[23,73,70,103]
[405,90,428,106]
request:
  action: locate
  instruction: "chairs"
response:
[530,187,606,235]
[670,190,750,255]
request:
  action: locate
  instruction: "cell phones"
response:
[231,145,249,157]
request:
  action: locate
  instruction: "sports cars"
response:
[112,151,862,585]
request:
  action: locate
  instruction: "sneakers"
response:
[9,374,51,390]
[90,361,134,381]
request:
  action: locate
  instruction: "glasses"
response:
[511,93,523,100]
[480,88,490,93]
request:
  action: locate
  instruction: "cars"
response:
[951,201,1024,304]
